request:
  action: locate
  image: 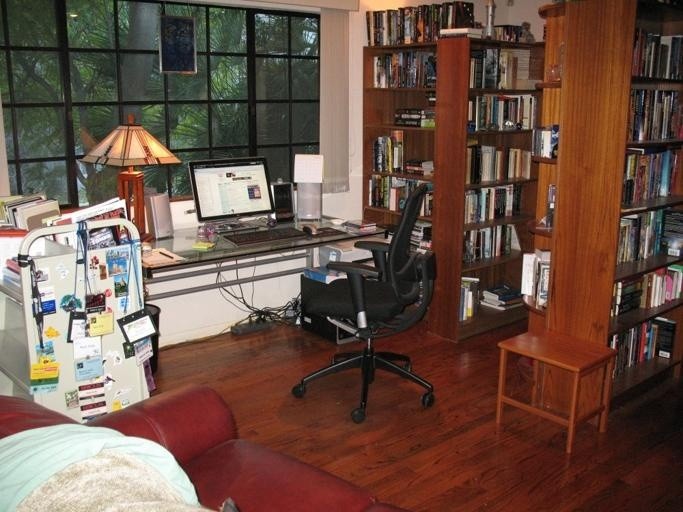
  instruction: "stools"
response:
[495,330,619,454]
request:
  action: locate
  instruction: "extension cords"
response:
[231,320,270,334]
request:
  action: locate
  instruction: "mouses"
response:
[303,223,318,235]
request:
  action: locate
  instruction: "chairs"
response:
[292,185,437,423]
[0,386,407,512]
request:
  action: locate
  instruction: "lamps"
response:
[79,114,182,244]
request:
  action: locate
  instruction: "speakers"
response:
[144,192,173,239]
[296,184,323,220]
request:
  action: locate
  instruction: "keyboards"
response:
[223,226,306,247]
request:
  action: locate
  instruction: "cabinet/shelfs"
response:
[1,220,143,420]
[522,1,683,429]
[363,42,436,337]
[426,35,544,344]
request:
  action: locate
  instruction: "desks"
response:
[142,214,385,304]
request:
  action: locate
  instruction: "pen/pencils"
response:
[158,252,173,260]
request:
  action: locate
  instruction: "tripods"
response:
[187,155,274,232]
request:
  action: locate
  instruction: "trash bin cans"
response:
[144,304,161,373]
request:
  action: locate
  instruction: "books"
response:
[349,225,377,232]
[611,24,683,390]
[366,107,435,249]
[365,3,539,92]
[0,192,158,423]
[481,283,524,312]
[140,248,185,269]
[546,185,555,233]
[520,251,551,310]
[464,92,538,186]
[532,125,558,160]
[345,219,377,229]
[459,276,480,321]
[465,184,521,264]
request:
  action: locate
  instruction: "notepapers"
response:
[193,242,214,249]
